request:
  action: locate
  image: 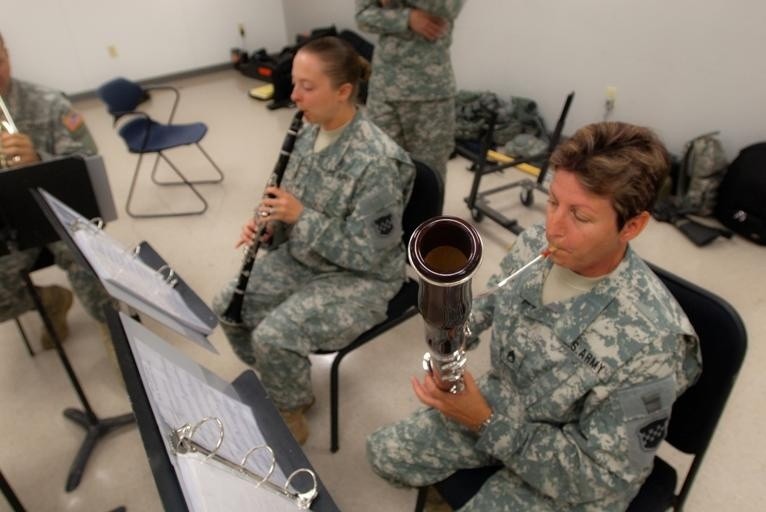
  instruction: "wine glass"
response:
[269,207,273,214]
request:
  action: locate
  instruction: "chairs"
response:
[32,287,72,350]
[279,395,315,445]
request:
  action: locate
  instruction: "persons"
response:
[363,122,705,512]
[211,35,418,448]
[0,35,122,349]
[355,0,462,190]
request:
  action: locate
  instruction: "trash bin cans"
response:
[478,414,493,436]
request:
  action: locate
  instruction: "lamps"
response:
[413,258,749,512]
[14,248,142,356]
[307,159,446,454]
[94,77,224,219]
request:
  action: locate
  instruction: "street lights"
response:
[454,90,551,158]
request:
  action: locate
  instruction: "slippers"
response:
[667,136,766,247]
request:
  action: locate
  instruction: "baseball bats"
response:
[651,197,721,248]
